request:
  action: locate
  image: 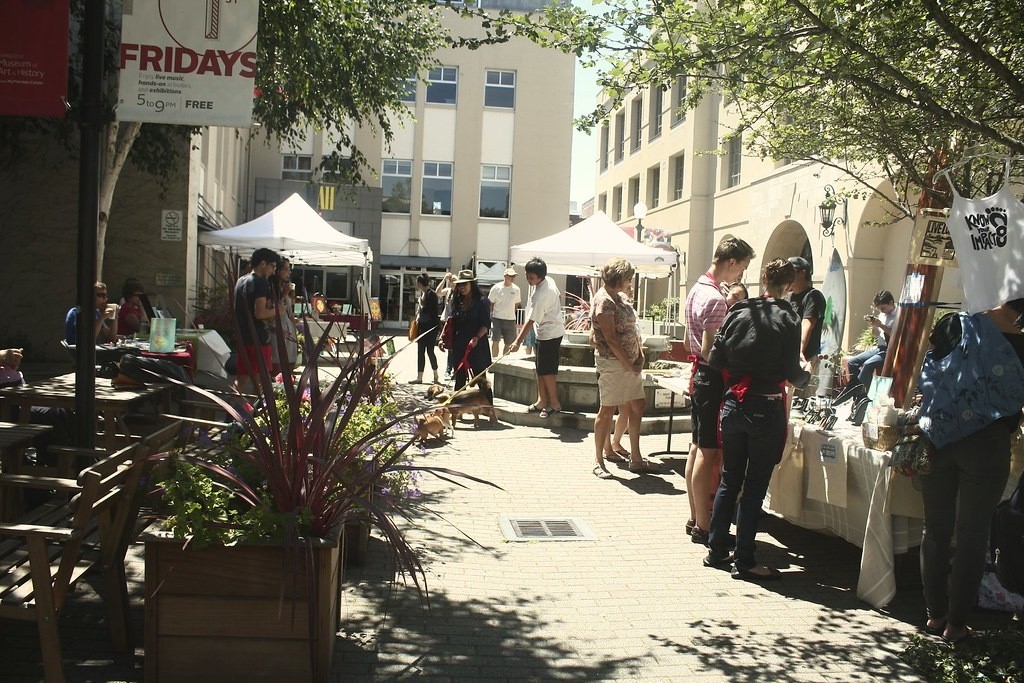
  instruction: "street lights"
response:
[633,200,649,315]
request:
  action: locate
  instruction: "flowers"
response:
[196,335,425,513]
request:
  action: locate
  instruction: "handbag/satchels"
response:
[408,320,419,341]
[440,315,454,349]
[889,424,938,476]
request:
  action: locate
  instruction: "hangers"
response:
[933,137,1024,185]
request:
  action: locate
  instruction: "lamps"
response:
[819,183,848,236]
[671,245,686,267]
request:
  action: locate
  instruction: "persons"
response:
[65,281,119,345]
[233,249,298,401]
[438,269,491,392]
[435,272,458,378]
[682,236,828,581]
[831,288,900,423]
[916,297,1024,645]
[508,257,566,418]
[589,258,661,479]
[117,287,148,335]
[488,268,521,357]
[408,273,439,384]
[0,347,77,466]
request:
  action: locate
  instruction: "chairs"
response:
[0,338,258,683]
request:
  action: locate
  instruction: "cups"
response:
[107,304,118,320]
[150,318,177,353]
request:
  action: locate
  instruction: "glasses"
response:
[96,293,108,298]
[457,283,468,286]
[281,267,291,272]
[270,263,277,271]
[510,276,515,277]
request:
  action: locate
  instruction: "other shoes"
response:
[690,526,709,544]
[408,381,422,384]
[685,519,696,535]
[731,567,782,580]
[433,376,439,384]
[703,552,734,567]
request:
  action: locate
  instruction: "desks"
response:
[0,372,174,464]
[640,370,926,609]
[95,328,231,381]
[297,313,379,341]
[0,422,51,520]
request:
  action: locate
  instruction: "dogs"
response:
[424,378,499,429]
[411,408,450,447]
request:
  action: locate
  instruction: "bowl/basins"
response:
[565,330,592,344]
[642,335,670,348]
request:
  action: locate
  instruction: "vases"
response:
[240,453,378,566]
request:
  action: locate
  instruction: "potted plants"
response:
[131,276,504,683]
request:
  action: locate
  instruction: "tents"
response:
[509,211,678,339]
[197,192,375,319]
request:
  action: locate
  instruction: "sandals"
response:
[628,458,661,473]
[593,463,613,478]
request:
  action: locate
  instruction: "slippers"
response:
[540,406,561,418]
[615,450,631,457]
[925,619,944,634]
[524,404,542,414]
[603,453,622,462]
[941,626,971,641]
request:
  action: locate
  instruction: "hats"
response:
[453,270,478,283]
[504,268,518,276]
